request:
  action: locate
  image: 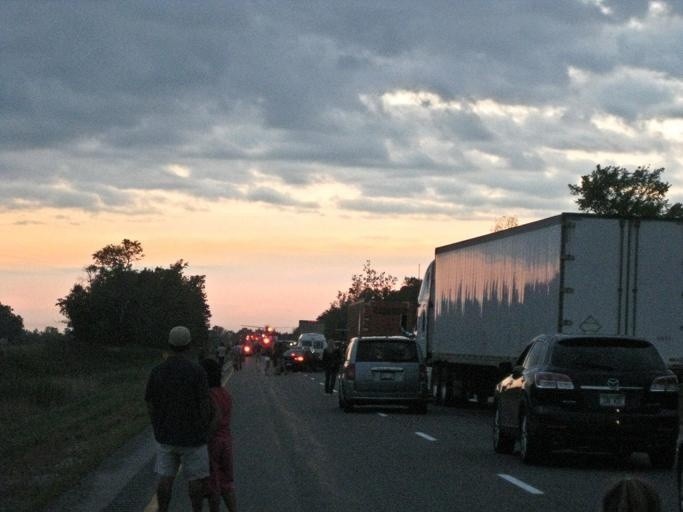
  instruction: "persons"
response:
[143,325,211,510]
[215,338,291,377]
[321,338,339,394]
[198,358,238,512]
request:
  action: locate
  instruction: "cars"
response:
[239,333,320,372]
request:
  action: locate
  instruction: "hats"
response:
[169,326,192,347]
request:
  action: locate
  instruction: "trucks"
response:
[399,213,683,407]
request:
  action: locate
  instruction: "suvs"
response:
[492,334,680,468]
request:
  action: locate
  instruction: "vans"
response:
[298,332,328,360]
[338,335,428,415]
